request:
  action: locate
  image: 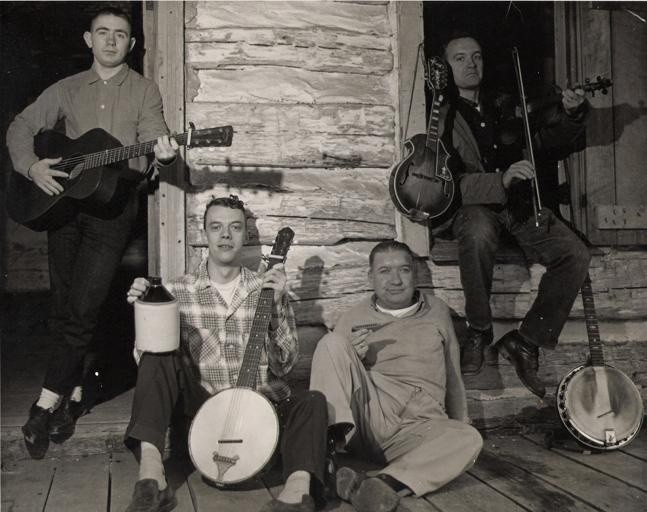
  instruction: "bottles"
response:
[133,278,182,353]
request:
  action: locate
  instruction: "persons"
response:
[437,34,597,400]
[125,196,330,512]
[309,238,484,512]
[4,8,191,449]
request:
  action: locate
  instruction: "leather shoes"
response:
[125,480,177,511]
[326,447,399,511]
[261,493,315,512]
[48,397,85,443]
[460,325,494,376]
[494,328,546,399]
[23,398,52,444]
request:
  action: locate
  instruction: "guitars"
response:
[3,123,234,229]
[388,55,456,225]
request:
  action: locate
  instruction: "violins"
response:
[489,77,614,134]
